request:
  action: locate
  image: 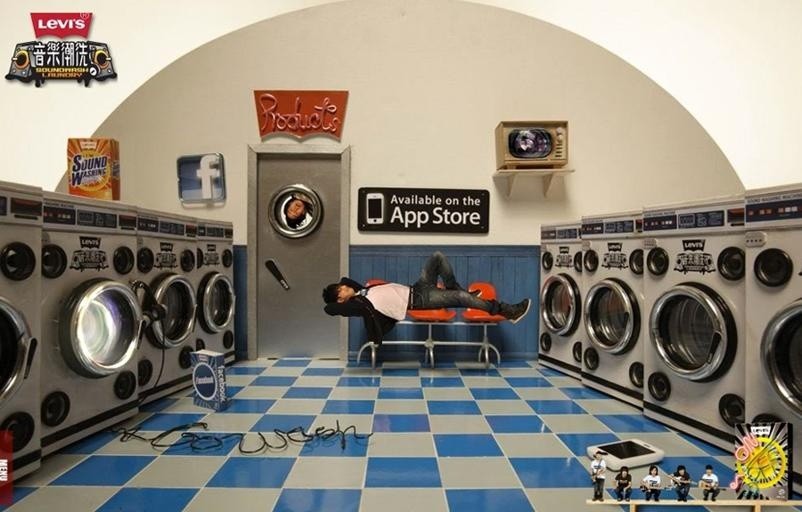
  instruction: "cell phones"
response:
[366,191,386,225]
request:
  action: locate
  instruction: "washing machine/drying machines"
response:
[581,212,644,410]
[41,189,139,459]
[200,218,236,372]
[538,222,581,380]
[643,194,746,455]
[138,204,200,408]
[746,182,802,496]
[0,180,41,482]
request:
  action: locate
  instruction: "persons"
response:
[589,450,607,501]
[701,465,720,501]
[322,251,531,346]
[613,466,632,501]
[642,465,662,501]
[669,465,690,501]
[283,190,316,229]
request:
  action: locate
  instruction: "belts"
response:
[410,287,414,309]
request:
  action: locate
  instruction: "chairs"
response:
[406,284,458,370]
[357,280,391,372]
[462,282,501,371]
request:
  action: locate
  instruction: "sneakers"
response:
[507,298,532,325]
[469,290,482,298]
